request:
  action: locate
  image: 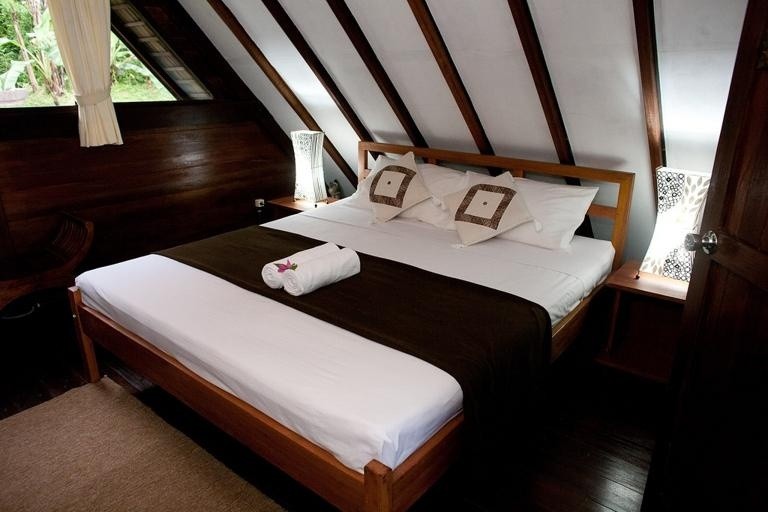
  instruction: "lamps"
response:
[289,130,329,202]
[636,165,712,283]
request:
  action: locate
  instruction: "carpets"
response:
[2,370,284,511]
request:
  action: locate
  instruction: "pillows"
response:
[350,149,602,253]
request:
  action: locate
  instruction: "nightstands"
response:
[266,196,341,222]
[591,260,695,383]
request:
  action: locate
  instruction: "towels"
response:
[261,240,362,299]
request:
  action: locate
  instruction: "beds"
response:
[65,139,638,512]
